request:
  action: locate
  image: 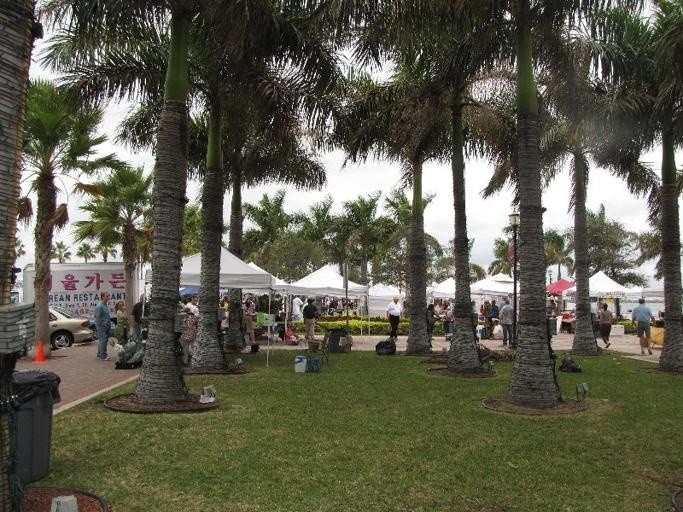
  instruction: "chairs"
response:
[304,333,330,361]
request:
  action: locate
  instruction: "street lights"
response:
[507,208,520,325]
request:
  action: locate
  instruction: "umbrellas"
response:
[545,277,576,297]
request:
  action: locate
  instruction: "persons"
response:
[92,291,117,362]
[128,288,616,351]
[627,295,658,355]
[110,298,129,344]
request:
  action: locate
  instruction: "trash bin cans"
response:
[327,329,352,353]
[549,318,557,335]
[11,369,60,484]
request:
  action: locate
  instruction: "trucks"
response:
[9,263,146,340]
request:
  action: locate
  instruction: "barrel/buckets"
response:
[308,341,320,354]
[294,355,307,373]
[307,358,320,372]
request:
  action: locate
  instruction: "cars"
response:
[47,305,98,350]
